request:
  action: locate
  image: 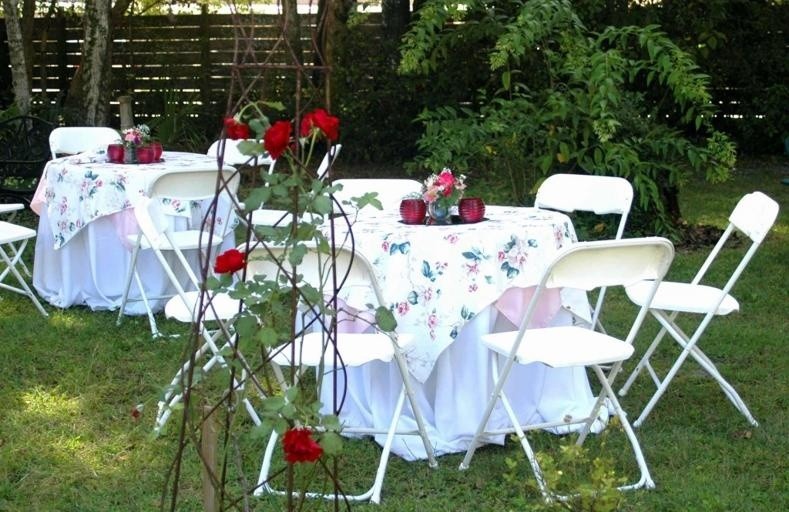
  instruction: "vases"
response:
[426,201,452,226]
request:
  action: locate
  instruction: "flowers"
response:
[190,95,397,511]
[423,169,468,206]
[122,124,154,145]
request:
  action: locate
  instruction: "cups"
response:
[105,142,164,164]
[457,197,487,224]
[399,198,429,224]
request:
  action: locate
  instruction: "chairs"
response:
[532,171,635,393]
[617,188,780,430]
[456,233,681,504]
[48,124,124,161]
[232,242,439,508]
[206,137,277,241]
[323,178,425,212]
[230,142,342,242]
[133,193,270,442]
[0,201,50,318]
[115,166,243,339]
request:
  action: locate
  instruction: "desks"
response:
[28,148,242,316]
[292,203,612,464]
